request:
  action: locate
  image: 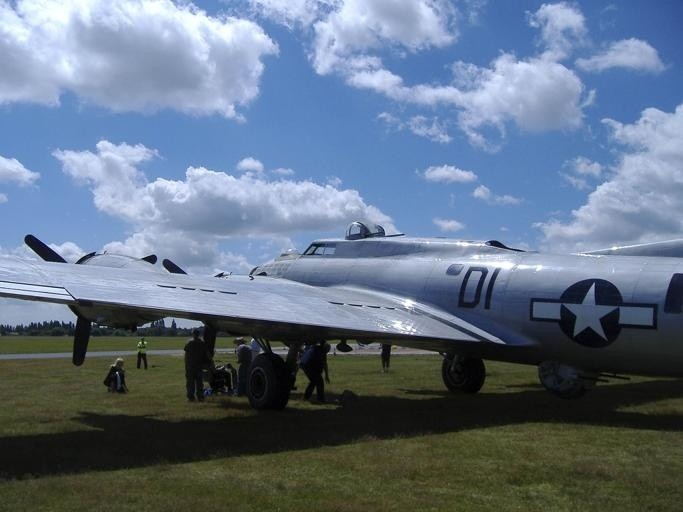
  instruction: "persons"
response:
[233,337,252,397]
[182,329,207,402]
[200,362,237,396]
[103,357,129,394]
[298,341,331,404]
[135,336,148,369]
[378,343,392,374]
[250,336,260,363]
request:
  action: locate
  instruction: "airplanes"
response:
[0,218,682,412]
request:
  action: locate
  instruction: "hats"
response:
[233,336,248,345]
